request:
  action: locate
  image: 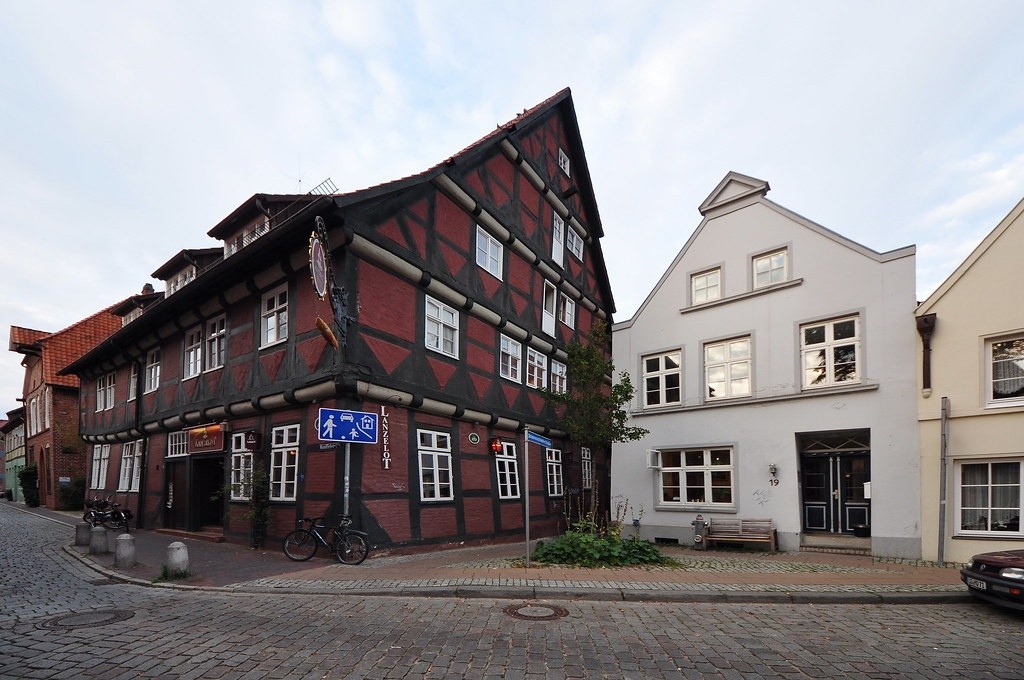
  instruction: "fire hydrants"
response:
[691,514,708,551]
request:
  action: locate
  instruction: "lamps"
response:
[770,464,777,474]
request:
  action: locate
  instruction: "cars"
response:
[959,549,1024,611]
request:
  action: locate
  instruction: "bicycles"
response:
[83,493,134,532]
[282,514,373,565]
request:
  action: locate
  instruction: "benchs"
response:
[700,517,777,554]
